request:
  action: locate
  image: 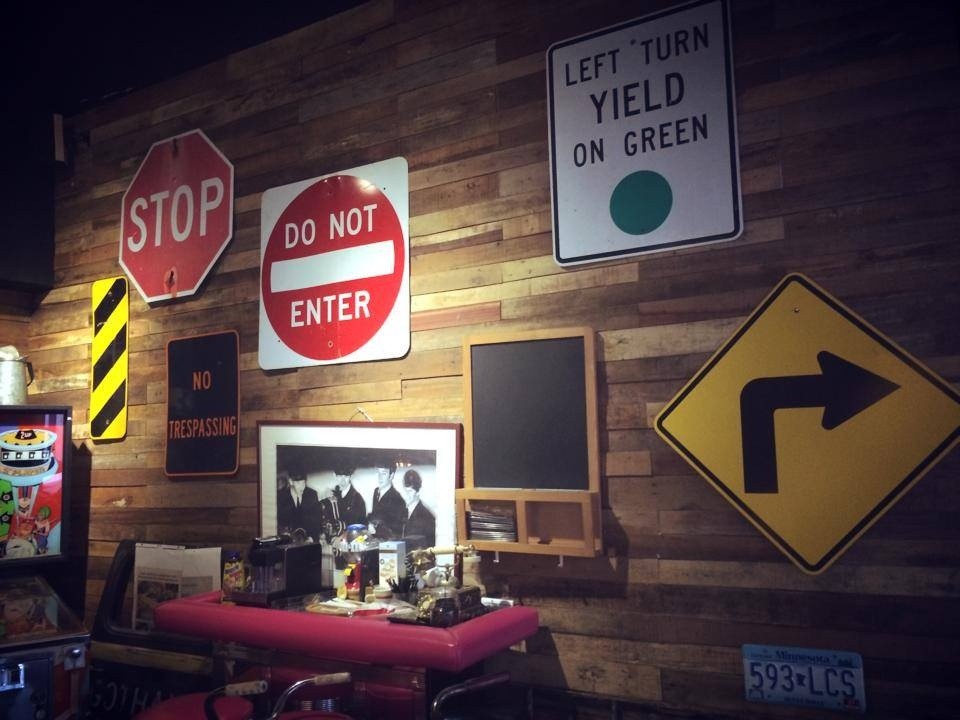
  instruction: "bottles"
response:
[220,551,245,606]
[347,524,366,549]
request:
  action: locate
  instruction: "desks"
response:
[151,586,540,720]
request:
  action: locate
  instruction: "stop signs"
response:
[262,175,405,361]
[116,128,236,304]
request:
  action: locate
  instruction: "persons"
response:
[332,460,365,526]
[372,458,406,535]
[401,469,435,555]
[277,462,318,543]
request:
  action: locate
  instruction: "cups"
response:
[373,588,393,605]
[391,592,417,606]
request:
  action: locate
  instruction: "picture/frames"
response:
[256,419,466,592]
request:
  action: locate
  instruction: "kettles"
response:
[0,345,34,405]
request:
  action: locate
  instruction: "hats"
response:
[332,464,355,477]
[404,470,422,488]
[289,470,306,481]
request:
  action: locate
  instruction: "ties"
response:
[298,495,300,508]
[378,492,380,500]
